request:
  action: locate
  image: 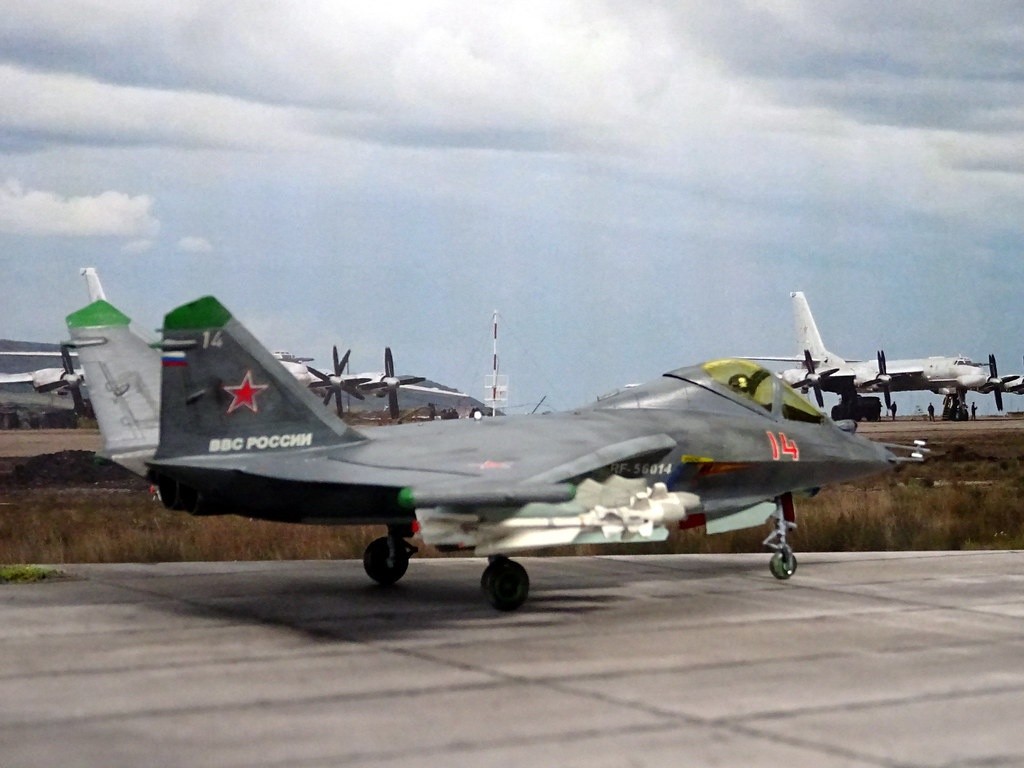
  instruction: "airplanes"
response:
[66,297,944,612]
[1,325,475,426]
[731,292,1024,431]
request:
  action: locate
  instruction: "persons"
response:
[927,402,936,422]
[969,402,978,421]
[890,401,898,421]
